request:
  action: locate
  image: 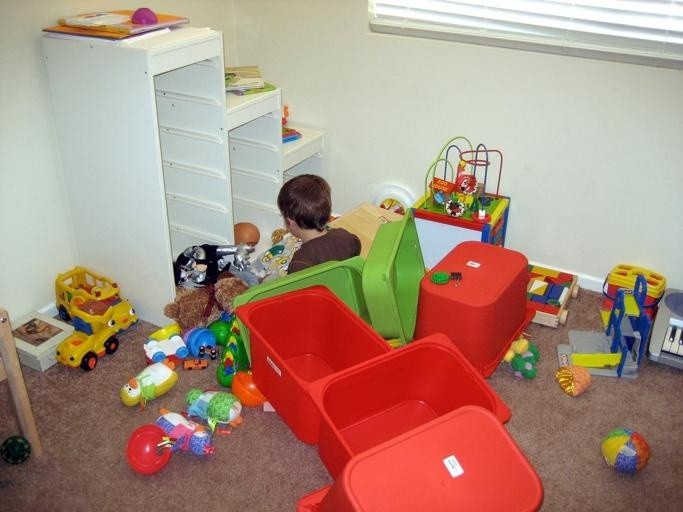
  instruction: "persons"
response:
[278,174,360,272]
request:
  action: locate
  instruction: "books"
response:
[40,9,189,46]
[224,64,265,92]
[12,311,76,374]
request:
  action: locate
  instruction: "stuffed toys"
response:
[120,358,177,410]
[165,272,248,331]
[186,388,242,428]
[156,407,213,455]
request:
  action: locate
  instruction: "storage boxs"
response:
[236,285,395,446]
[233,255,373,366]
[294,405,544,512]
[412,241,533,379]
[308,333,511,479]
[362,208,426,345]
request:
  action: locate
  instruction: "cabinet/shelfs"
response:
[41,25,325,330]
